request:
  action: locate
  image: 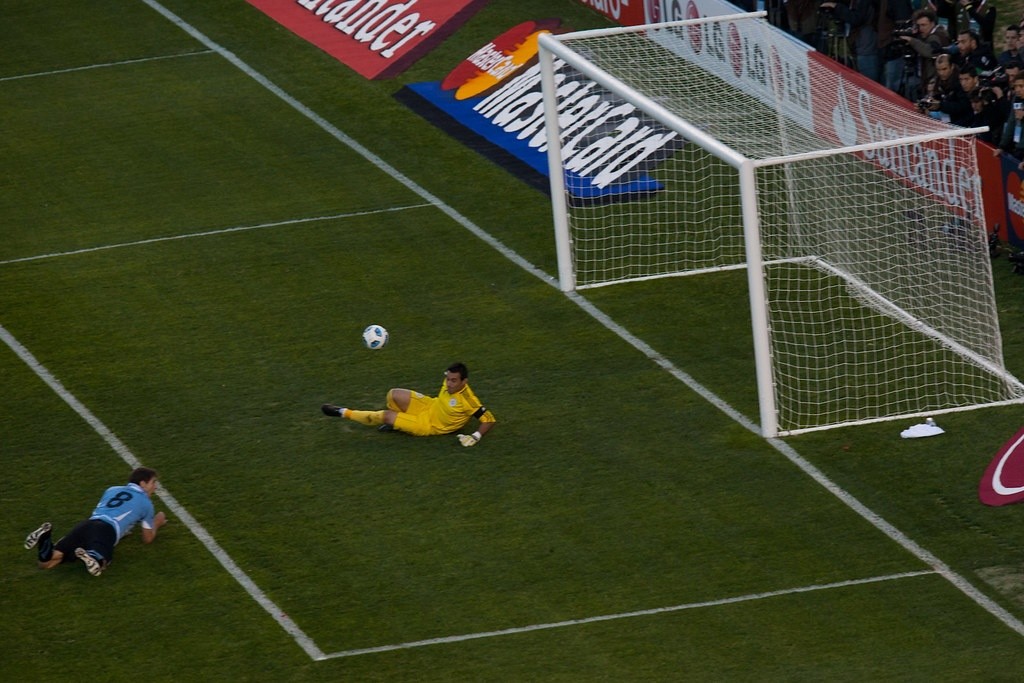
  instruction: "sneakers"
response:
[321,404,342,417]
[377,424,399,433]
[74,547,102,577]
[24,522,52,550]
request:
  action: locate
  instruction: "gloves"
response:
[456,430,482,447]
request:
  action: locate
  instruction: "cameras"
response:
[917,98,934,110]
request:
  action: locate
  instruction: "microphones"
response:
[1013,95,1024,110]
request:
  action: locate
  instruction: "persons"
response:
[885,1,1023,163]
[23,465,166,577]
[320,363,497,448]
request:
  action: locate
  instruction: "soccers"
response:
[362,325,390,351]
